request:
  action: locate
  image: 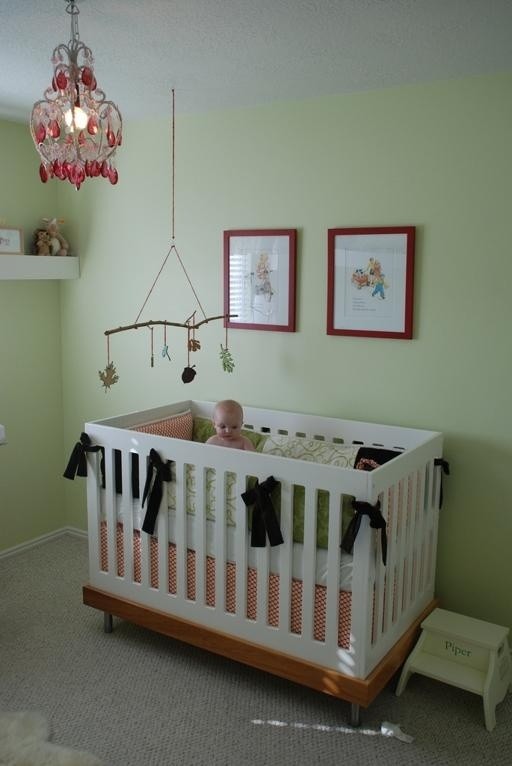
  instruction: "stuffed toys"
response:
[29,218,69,256]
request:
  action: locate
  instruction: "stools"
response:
[396,603,509,732]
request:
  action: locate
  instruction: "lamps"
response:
[27,0,123,188]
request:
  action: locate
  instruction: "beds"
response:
[76,395,443,727]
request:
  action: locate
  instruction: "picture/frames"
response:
[221,229,303,332]
[1,224,25,255]
[325,227,419,341]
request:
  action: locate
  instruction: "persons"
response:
[204,399,256,451]
[364,257,388,299]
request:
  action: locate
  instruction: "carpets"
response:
[0,707,104,766]
